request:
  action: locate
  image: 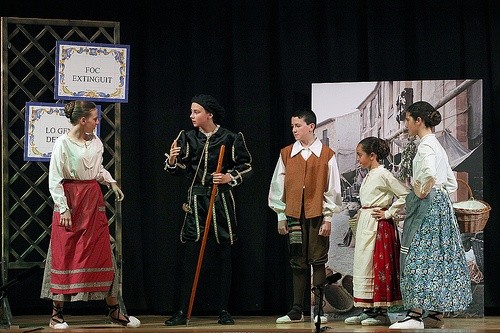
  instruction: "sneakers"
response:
[218,311,234,324]
[165,310,190,326]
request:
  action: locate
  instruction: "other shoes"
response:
[389,310,425,330]
[49,306,69,330]
[345,308,390,325]
[422,311,445,329]
[313,305,328,324]
[276,304,304,324]
[105,303,141,328]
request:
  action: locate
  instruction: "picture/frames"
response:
[54,41,130,102]
[24,102,102,161]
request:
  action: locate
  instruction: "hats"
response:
[191,94,221,115]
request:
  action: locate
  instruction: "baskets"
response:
[321,283,354,313]
[349,219,358,238]
[472,232,484,274]
[449,178,491,234]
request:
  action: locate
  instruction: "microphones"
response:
[312,273,342,290]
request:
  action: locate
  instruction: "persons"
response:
[389,100,459,330]
[48,100,140,330]
[268,108,337,324]
[344,136,409,325]
[159,95,255,326]
